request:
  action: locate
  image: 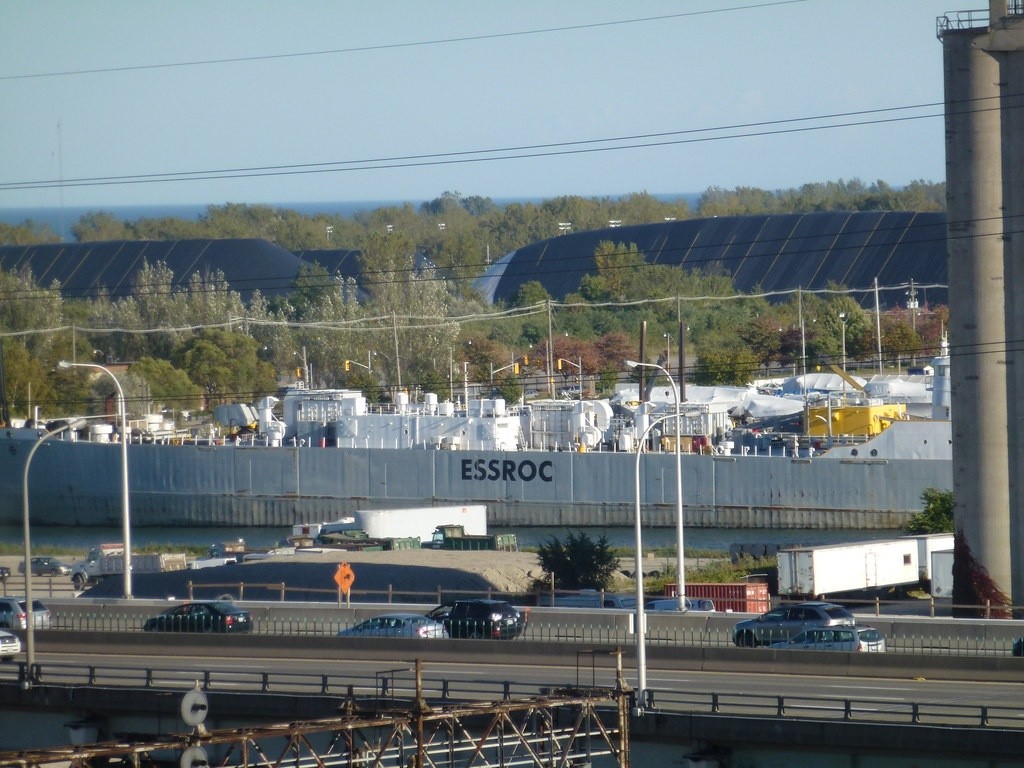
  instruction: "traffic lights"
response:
[575,378,579,384]
[345,360,349,371]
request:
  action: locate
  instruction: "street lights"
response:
[449,340,472,402]
[22,419,88,675]
[367,350,377,376]
[293,346,310,389]
[842,317,848,397]
[635,409,703,703]
[624,360,687,611]
[545,332,569,396]
[58,360,133,601]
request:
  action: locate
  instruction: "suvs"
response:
[731,601,856,647]
[423,599,523,639]
[0,596,52,631]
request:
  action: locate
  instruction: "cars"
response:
[645,598,715,611]
[143,601,253,633]
[18,556,57,574]
[34,561,72,576]
[525,390,539,399]
[756,383,784,395]
[0,567,11,581]
[336,613,450,639]
[765,627,886,653]
[0,631,21,661]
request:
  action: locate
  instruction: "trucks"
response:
[71,543,188,592]
[189,502,519,572]
[645,582,769,613]
